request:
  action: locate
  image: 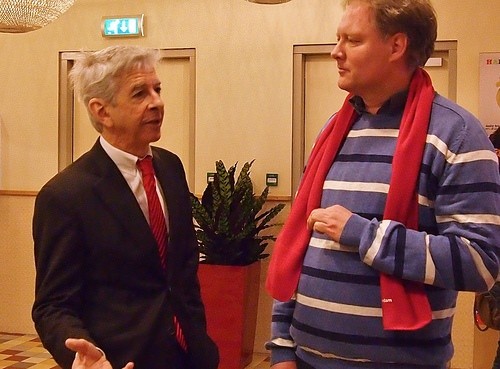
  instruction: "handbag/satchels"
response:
[474,281,500,331]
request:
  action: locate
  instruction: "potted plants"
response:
[187,157,287,369]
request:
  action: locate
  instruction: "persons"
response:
[32,45,219,369]
[265,0,500,369]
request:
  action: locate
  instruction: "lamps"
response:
[0,0,74,32]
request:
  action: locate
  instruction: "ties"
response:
[136,156,188,350]
